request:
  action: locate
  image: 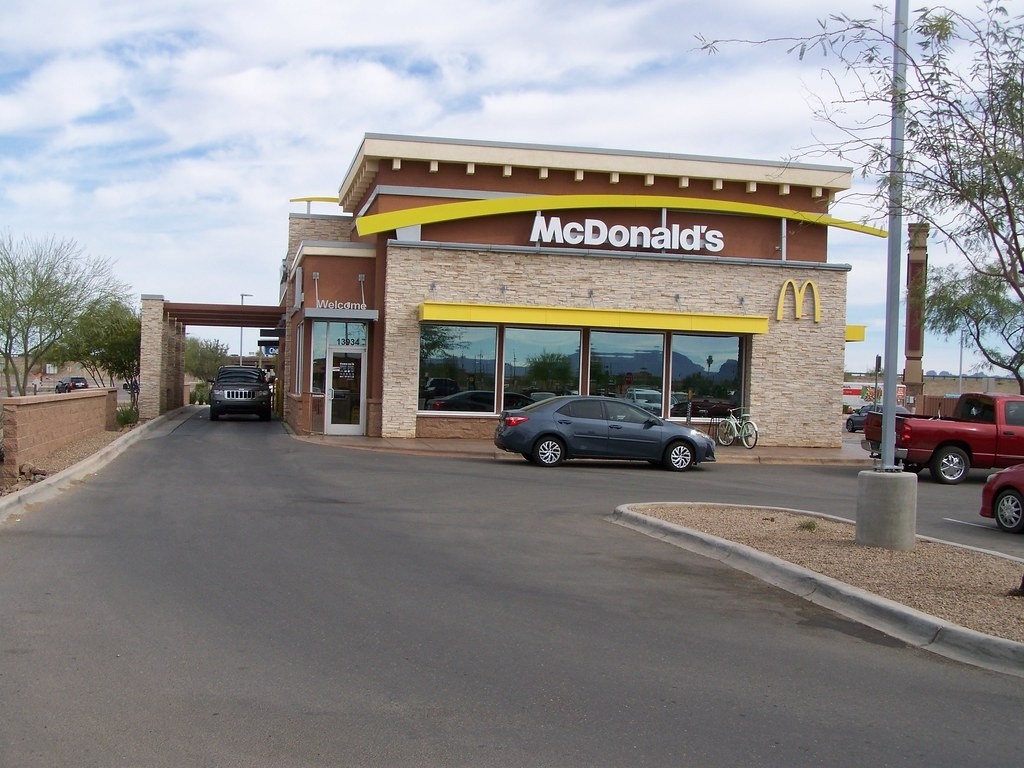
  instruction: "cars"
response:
[420,379,460,400]
[846,403,910,432]
[427,390,540,413]
[979,463,1024,535]
[529,388,741,417]
[493,395,717,472]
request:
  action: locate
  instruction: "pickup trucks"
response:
[861,392,1024,486]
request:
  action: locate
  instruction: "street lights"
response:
[239,293,254,366]
[959,329,969,394]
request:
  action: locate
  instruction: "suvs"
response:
[206,365,275,422]
[55,376,89,394]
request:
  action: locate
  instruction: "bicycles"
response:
[717,406,759,449]
